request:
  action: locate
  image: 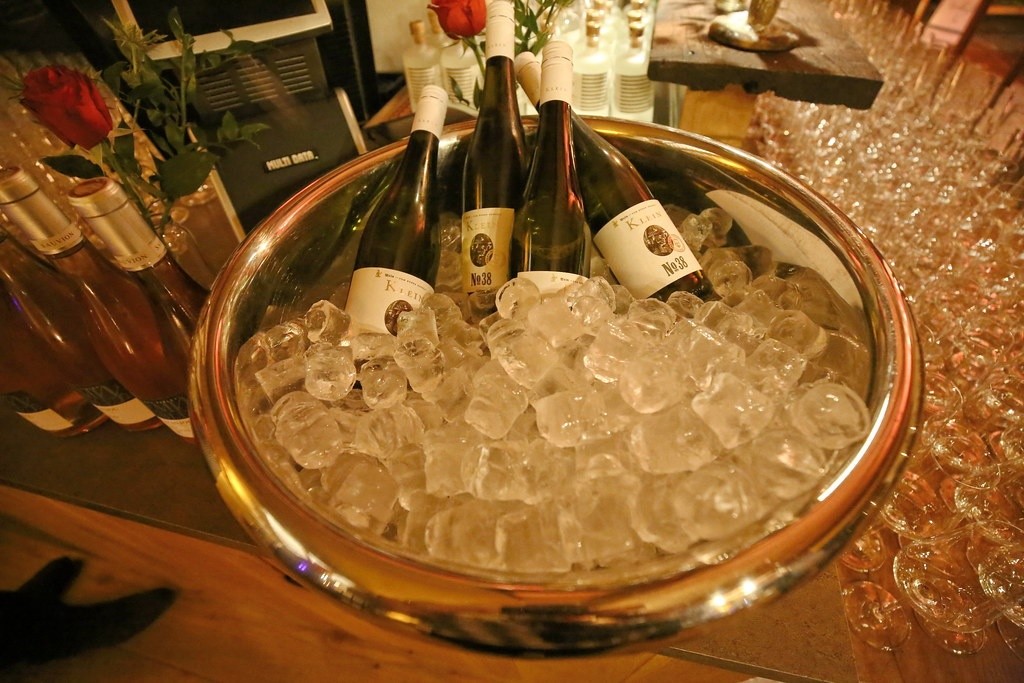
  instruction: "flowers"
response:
[15,4,269,229]
[418,0,574,104]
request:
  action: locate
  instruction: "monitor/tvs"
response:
[111,0,334,63]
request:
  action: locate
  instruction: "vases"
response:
[143,213,219,289]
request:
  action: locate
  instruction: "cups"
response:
[713,0,746,12]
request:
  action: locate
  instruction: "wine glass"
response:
[742,0,1024,659]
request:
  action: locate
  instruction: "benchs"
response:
[642,1,884,151]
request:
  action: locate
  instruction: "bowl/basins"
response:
[185,114,926,663]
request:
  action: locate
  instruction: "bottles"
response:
[400,9,483,114]
[461,0,530,328]
[0,165,203,446]
[572,0,658,125]
[344,84,451,338]
[513,40,715,305]
